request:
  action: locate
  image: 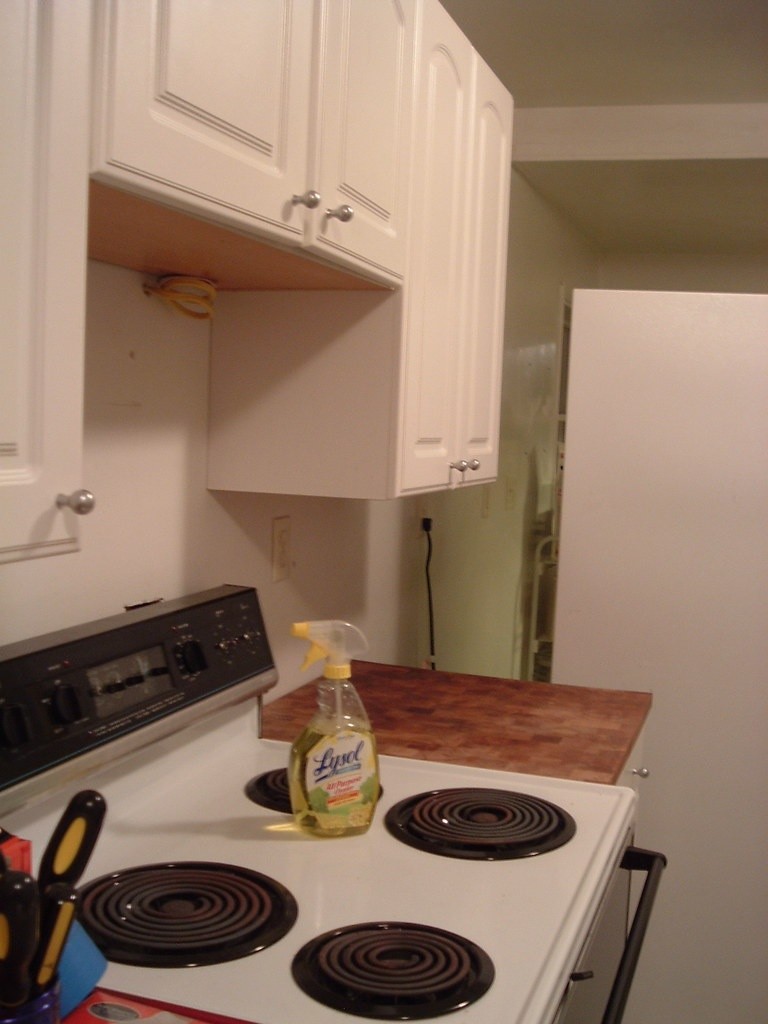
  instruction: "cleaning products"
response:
[287,620,380,840]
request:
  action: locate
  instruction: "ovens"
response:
[549,825,668,1024]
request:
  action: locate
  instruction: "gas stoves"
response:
[0,583,635,1024]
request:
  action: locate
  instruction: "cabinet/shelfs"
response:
[206,0,514,500]
[0,0,95,563]
[88,0,416,291]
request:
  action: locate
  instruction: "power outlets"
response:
[415,494,433,539]
[271,515,292,584]
